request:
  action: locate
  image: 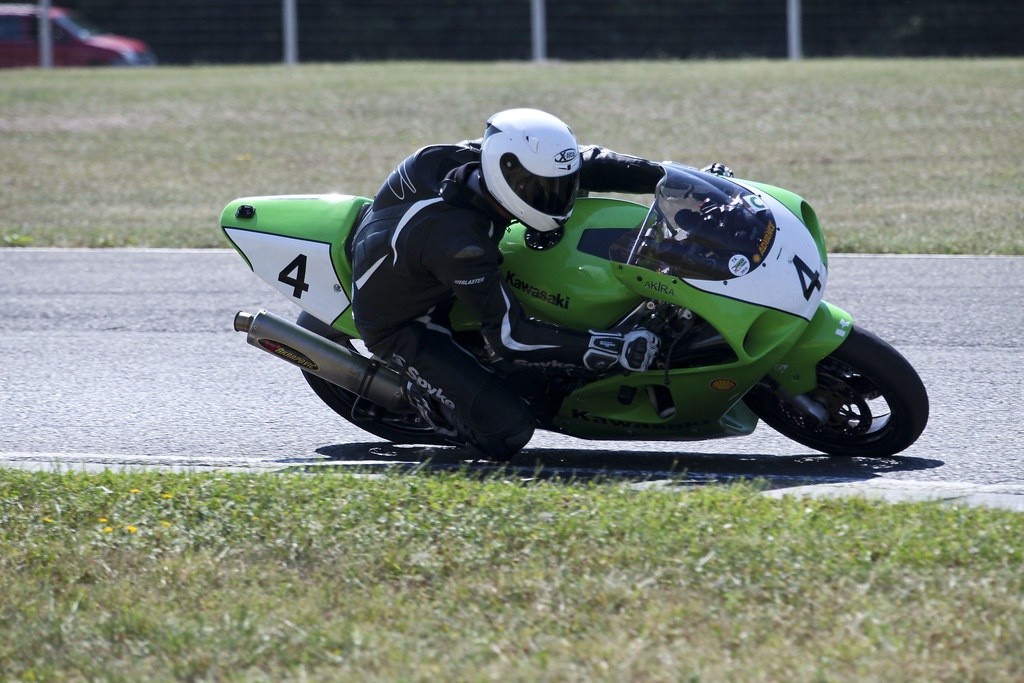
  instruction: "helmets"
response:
[481,108,582,232]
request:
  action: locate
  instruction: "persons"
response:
[349,107,738,463]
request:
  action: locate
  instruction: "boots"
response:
[400,376,479,447]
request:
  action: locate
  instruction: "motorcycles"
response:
[218,160,930,459]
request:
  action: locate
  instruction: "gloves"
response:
[659,160,733,197]
[583,328,661,374]
